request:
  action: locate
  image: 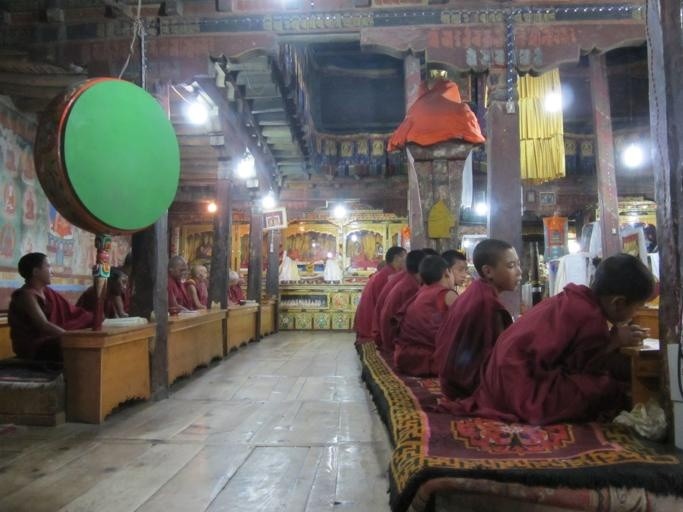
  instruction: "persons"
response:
[308,238,320,256]
[8,253,105,361]
[75,252,246,318]
[386,64,486,152]
[4,142,73,235]
[431,251,655,427]
[197,234,214,258]
[355,240,526,401]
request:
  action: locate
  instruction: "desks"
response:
[164,308,224,386]
[224,303,259,360]
[617,337,662,415]
[60,317,159,420]
[259,298,278,335]
